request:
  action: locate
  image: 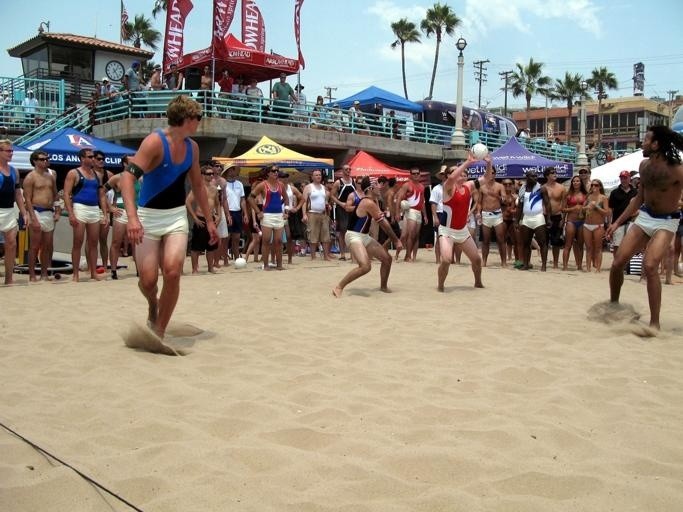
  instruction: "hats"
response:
[221,162,237,177]
[578,167,589,175]
[619,171,631,178]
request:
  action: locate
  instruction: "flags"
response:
[121,4,129,39]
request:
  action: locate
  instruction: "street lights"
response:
[573,77,589,167]
[448,32,472,147]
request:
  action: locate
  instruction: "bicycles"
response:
[594,145,616,167]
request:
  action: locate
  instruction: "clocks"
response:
[105,59,124,81]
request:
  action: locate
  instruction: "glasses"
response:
[270,169,279,173]
[204,165,222,177]
[85,155,105,162]
[0,149,13,153]
[36,158,49,161]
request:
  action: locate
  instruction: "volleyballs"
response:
[471,143,489,158]
[234,258,247,269]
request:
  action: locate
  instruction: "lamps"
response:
[36,19,50,33]
